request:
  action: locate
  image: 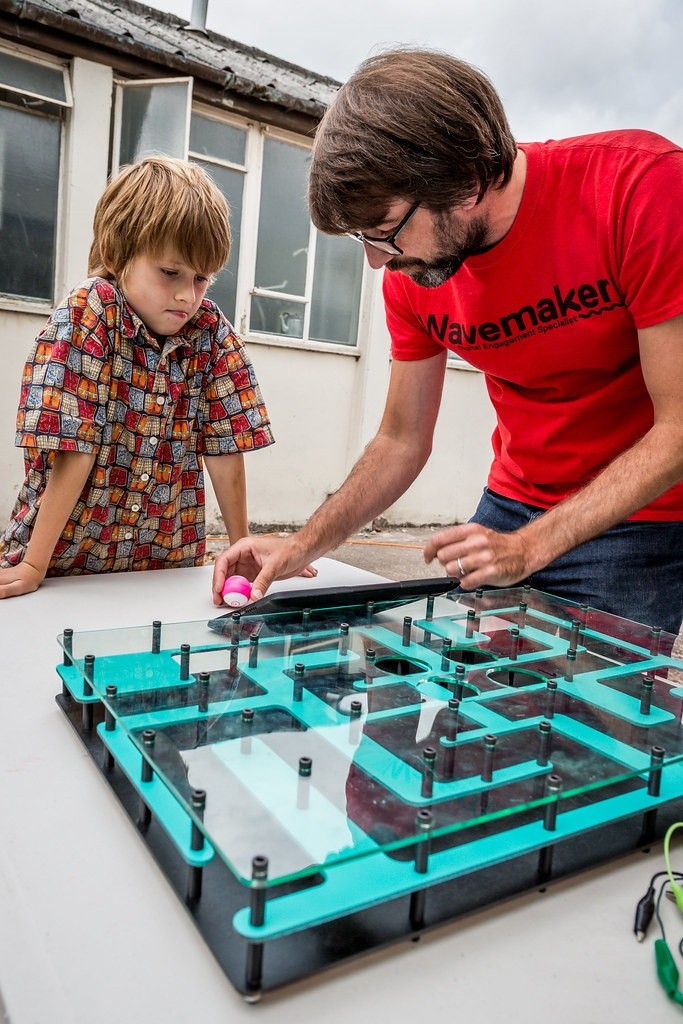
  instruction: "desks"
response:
[0,557,683,1024]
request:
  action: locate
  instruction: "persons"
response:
[212,52,683,679]
[1,160,318,599]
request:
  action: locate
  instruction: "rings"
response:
[457,559,468,580]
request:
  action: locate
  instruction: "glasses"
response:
[345,189,427,255]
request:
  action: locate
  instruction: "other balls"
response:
[221,574,252,607]
